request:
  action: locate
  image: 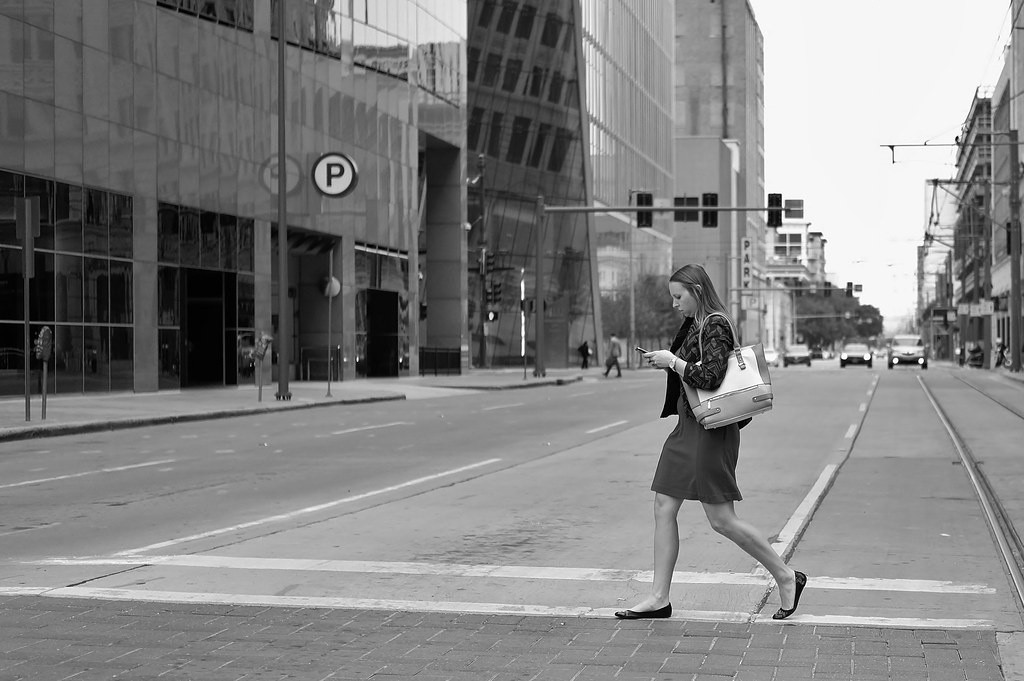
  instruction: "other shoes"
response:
[615,602,673,620]
[602,372,608,378]
[614,375,622,378]
[772,570,807,619]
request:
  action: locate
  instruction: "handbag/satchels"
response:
[679,312,773,430]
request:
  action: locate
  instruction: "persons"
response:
[577,341,592,369]
[614,264,807,619]
[601,333,622,378]
[777,335,788,367]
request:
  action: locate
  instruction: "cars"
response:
[781,344,812,368]
[764,349,780,367]
[811,346,822,359]
[840,342,874,368]
[876,352,884,358]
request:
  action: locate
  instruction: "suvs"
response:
[886,335,929,370]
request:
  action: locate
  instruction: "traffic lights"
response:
[485,288,492,303]
[491,280,502,305]
[483,251,495,276]
[487,310,498,321]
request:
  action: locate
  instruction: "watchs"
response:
[669,356,677,373]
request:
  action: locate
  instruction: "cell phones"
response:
[635,347,648,354]
